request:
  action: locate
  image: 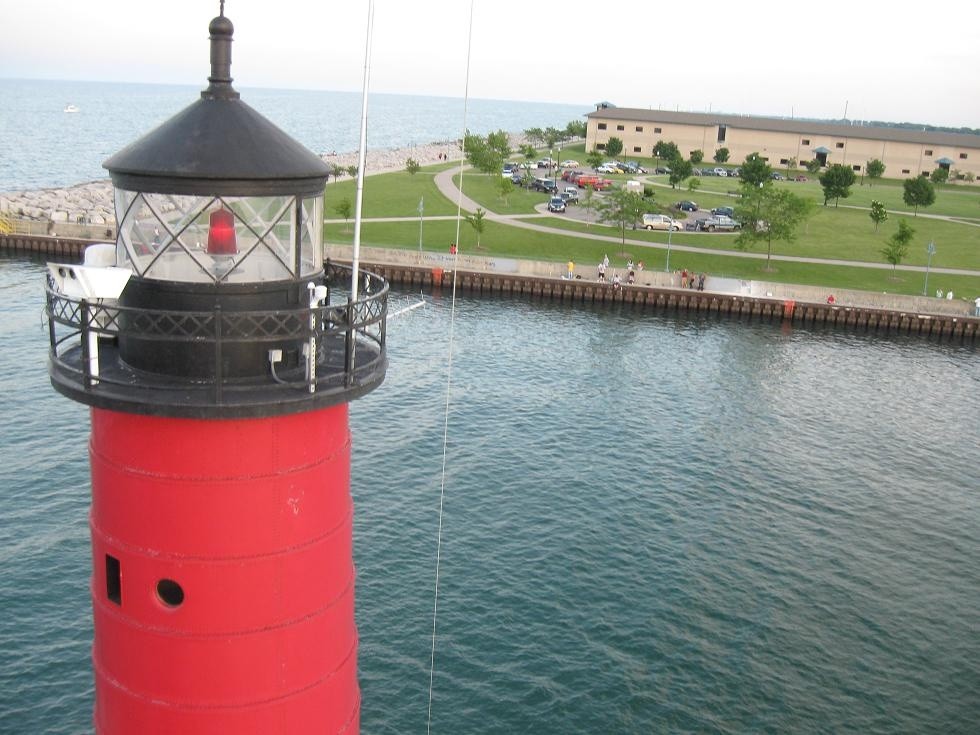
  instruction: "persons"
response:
[676,268,705,291]
[975,296,980,317]
[597,264,605,282]
[629,272,634,285]
[153,226,159,243]
[827,295,835,304]
[568,260,574,279]
[637,260,643,270]
[449,244,456,254]
[628,259,633,269]
[946,289,952,300]
[439,153,447,160]
[936,289,943,297]
[604,254,609,267]
[613,275,622,289]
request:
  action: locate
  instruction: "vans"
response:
[640,213,684,232]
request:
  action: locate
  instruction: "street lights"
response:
[549,149,553,177]
[557,147,561,172]
[554,166,558,197]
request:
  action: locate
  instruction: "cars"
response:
[711,206,735,219]
[685,215,742,234]
[547,196,566,213]
[655,165,809,183]
[501,156,649,204]
[676,200,698,211]
